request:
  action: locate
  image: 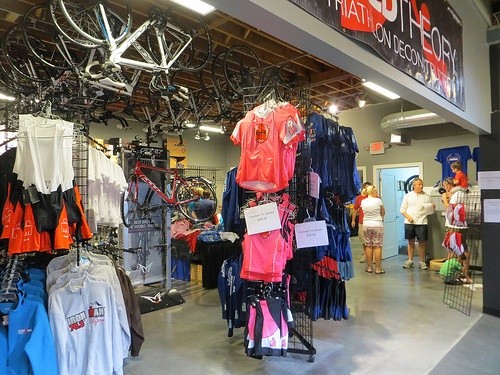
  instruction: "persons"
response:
[352,181,385,275]
[439,254,467,285]
[399,179,437,270]
[189,187,215,224]
[447,161,468,195]
[441,177,474,284]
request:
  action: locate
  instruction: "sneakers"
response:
[403,260,413,268]
[418,262,428,269]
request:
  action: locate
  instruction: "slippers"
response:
[366,267,373,273]
[375,269,385,274]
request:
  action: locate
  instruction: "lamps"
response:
[366,141,389,155]
[195,130,209,140]
[353,94,366,109]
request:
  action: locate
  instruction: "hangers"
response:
[0,101,136,316]
[239,191,299,219]
[242,89,296,121]
[247,280,285,305]
[302,158,339,230]
[300,101,353,130]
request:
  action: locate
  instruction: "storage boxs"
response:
[170,259,203,284]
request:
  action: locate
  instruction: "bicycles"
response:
[120,138,217,221]
[0,0,312,148]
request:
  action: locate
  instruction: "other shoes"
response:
[462,278,471,283]
[360,258,365,263]
[450,281,461,285]
